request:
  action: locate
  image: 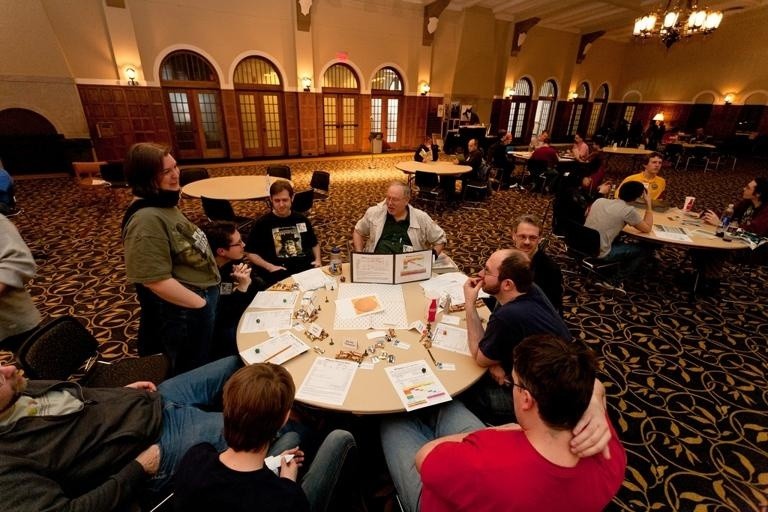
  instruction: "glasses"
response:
[484,267,497,278]
[230,239,242,247]
[503,374,533,398]
[515,232,541,241]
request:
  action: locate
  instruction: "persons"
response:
[243,181,322,283]
[583,153,666,287]
[0,159,21,217]
[460,247,576,416]
[120,143,221,375]
[527,129,604,200]
[377,334,627,512]
[202,220,265,356]
[511,212,562,315]
[352,183,446,262]
[686,128,706,158]
[613,119,665,151]
[414,131,514,201]
[1,364,293,512]
[690,176,767,272]
[174,361,359,512]
[0,212,40,353]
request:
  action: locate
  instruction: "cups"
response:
[682,196,697,213]
[424,291,442,324]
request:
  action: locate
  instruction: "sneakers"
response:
[595,279,628,298]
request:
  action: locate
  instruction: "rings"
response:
[240,269,243,273]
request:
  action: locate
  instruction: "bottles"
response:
[329,247,343,276]
[723,217,740,242]
[686,199,694,210]
[716,203,736,239]
[427,298,437,322]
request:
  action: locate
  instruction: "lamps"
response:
[303,77,313,92]
[631,1,725,53]
[420,84,432,96]
[125,66,140,87]
[568,90,579,102]
[504,88,514,100]
[724,97,733,104]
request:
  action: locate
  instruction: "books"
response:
[349,248,434,285]
